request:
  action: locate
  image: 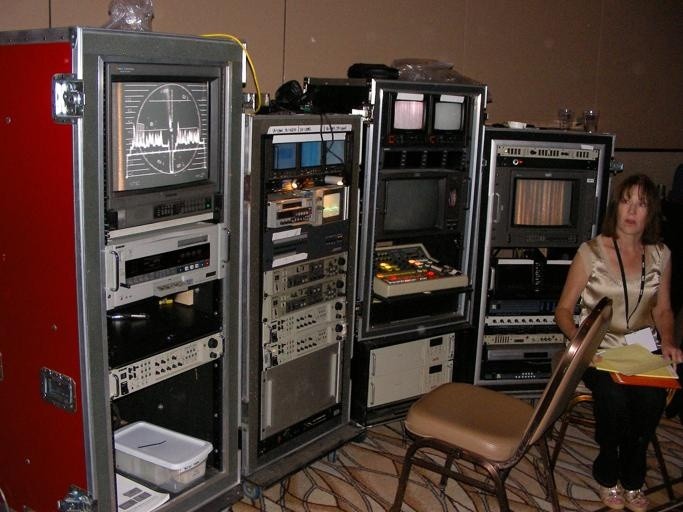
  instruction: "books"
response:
[589,345,682,392]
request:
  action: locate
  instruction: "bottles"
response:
[300,78,313,114]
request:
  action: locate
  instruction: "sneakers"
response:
[621,481,650,512]
[598,479,625,510]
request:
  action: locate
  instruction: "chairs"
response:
[389,297,613,512]
[548,380,682,504]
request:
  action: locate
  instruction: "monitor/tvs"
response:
[103,62,221,232]
[388,93,427,137]
[374,168,462,238]
[492,166,596,248]
[428,94,466,136]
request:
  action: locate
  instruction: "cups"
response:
[241,91,270,114]
[558,104,574,130]
[583,106,599,133]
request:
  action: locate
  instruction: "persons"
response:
[553,174,682,512]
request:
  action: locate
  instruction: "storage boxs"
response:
[113,420,213,494]
